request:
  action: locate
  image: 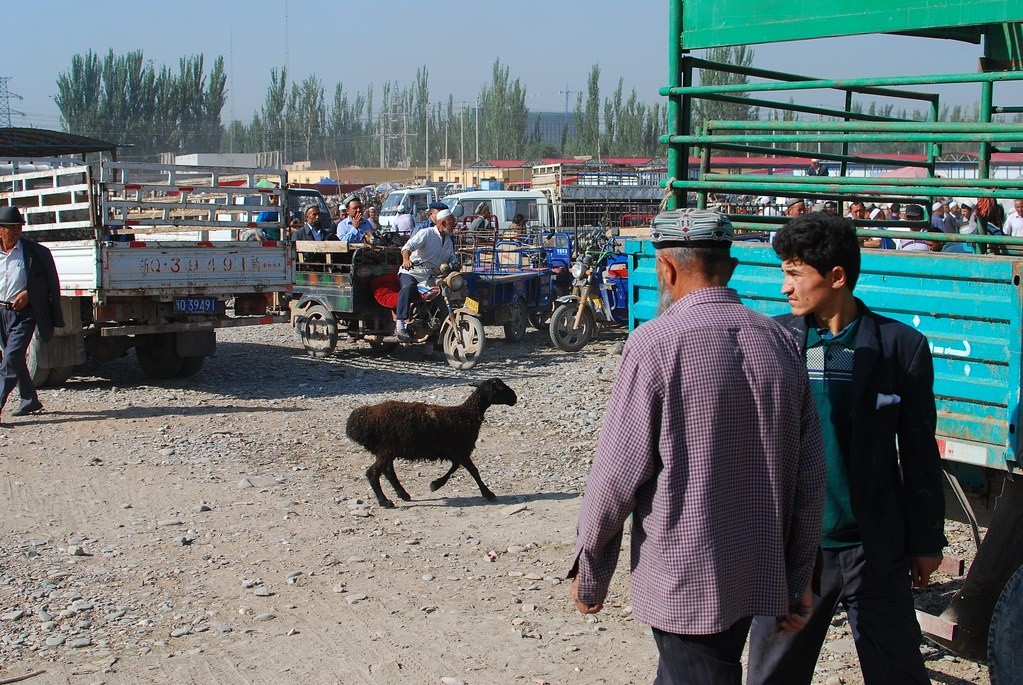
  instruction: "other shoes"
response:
[11,402,43,416]
[423,350,446,363]
[394,328,412,342]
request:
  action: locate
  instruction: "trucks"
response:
[375,185,456,244]
[148,187,339,245]
[0,124,297,390]
[441,181,697,237]
[621,1,1023,685]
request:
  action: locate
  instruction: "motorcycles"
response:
[449,230,632,353]
[286,244,487,373]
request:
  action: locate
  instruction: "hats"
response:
[429,202,449,209]
[949,202,957,210]
[962,199,974,209]
[869,208,881,219]
[437,209,454,222]
[824,199,837,206]
[397,204,404,213]
[475,202,485,214]
[932,203,943,212]
[339,205,347,211]
[849,201,855,209]
[906,205,923,217]
[303,203,319,216]
[650,208,733,250]
[342,194,357,206]
[812,158,819,164]
[756,196,771,206]
[368,207,375,211]
[787,198,804,208]
[0,206,26,225]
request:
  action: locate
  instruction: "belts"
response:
[1,303,13,310]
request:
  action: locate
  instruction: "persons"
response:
[393,205,416,236]
[565,207,828,685]
[255,187,385,274]
[0,206,66,416]
[715,211,949,685]
[410,201,449,239]
[465,202,491,243]
[502,213,525,244]
[394,209,460,343]
[706,156,1023,253]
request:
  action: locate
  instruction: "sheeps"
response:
[346,377,518,508]
